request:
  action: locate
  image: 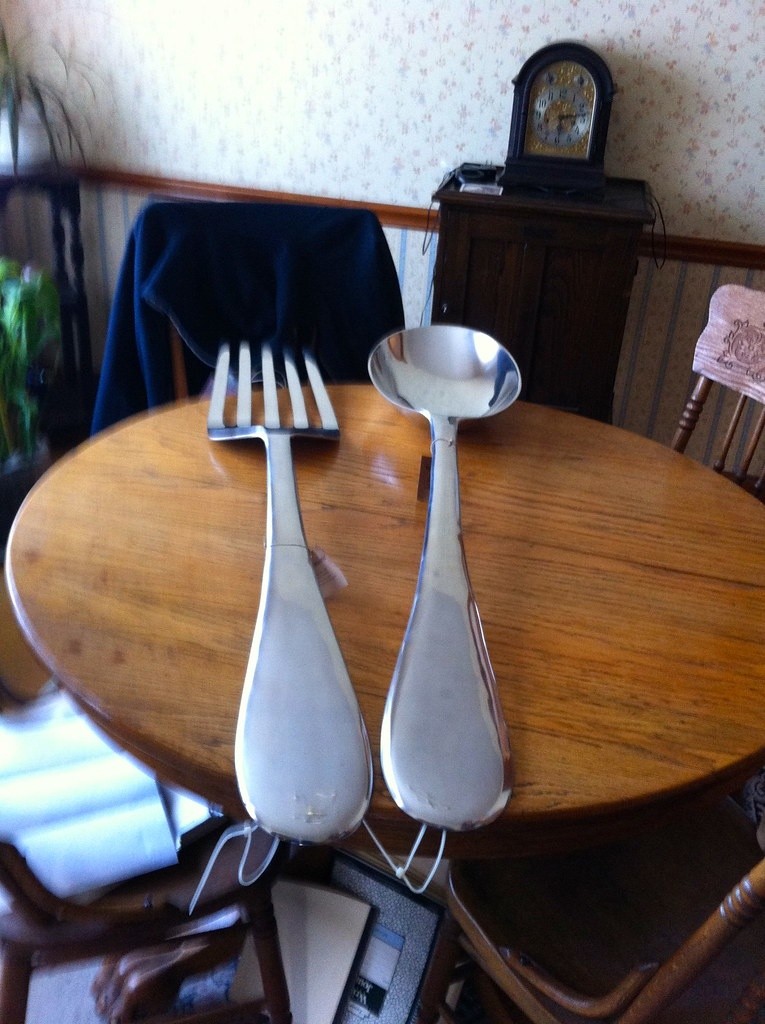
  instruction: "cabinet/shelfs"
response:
[431,163,654,423]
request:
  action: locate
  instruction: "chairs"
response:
[670,282,765,506]
[0,819,294,1024]
[94,196,399,399]
[411,796,765,1024]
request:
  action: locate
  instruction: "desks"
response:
[6,385,765,1024]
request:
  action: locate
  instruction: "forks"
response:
[207,342,376,844]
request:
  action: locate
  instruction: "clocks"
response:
[496,41,613,201]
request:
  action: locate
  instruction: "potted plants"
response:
[0,252,60,549]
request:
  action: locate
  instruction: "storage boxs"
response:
[229,874,372,1024]
